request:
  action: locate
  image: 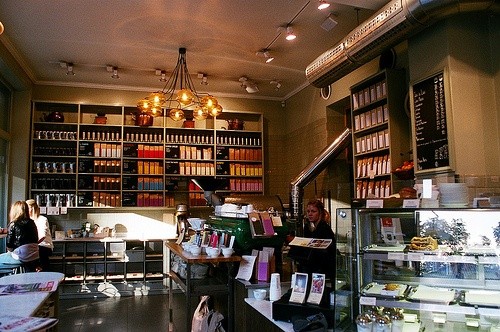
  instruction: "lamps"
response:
[59,61,78,79]
[256,0,331,64]
[239,77,249,90]
[107,66,121,80]
[270,80,283,91]
[136,48,224,122]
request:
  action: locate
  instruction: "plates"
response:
[437,182,470,208]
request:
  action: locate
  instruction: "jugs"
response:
[42,111,64,123]
[227,118,244,130]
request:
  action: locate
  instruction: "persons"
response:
[26,199,55,273]
[0,201,40,274]
[288,200,336,277]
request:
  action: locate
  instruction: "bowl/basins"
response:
[182,242,201,256]
[253,289,266,301]
[222,248,233,258]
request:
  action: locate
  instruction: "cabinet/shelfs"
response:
[356,202,500,331]
[48,236,178,282]
[27,99,263,209]
[343,66,410,203]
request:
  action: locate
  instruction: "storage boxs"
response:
[392,158,414,179]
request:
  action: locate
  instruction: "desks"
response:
[163,242,242,331]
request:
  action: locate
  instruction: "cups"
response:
[270,273,281,301]
[205,247,219,258]
